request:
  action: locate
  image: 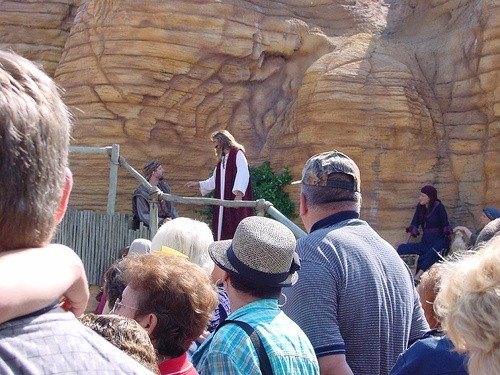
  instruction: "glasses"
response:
[113,298,140,313]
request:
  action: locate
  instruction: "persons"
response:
[132,159,178,231]
[0,50,155,375]
[191,216,320,375]
[78,217,232,375]
[278,150,431,375]
[109,252,219,375]
[0,244,90,323]
[396,185,454,287]
[184,130,257,241]
[390,207,500,375]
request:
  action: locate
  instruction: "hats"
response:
[210,216,301,286]
[291,151,360,192]
[143,160,160,174]
